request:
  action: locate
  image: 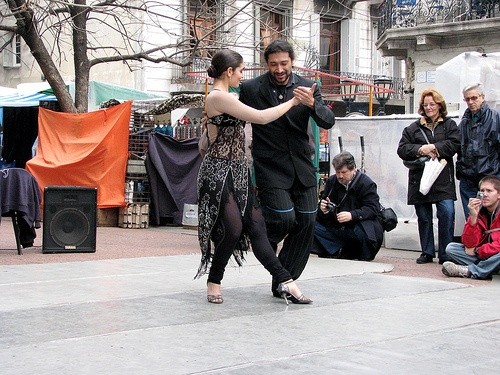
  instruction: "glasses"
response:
[422,102,438,107]
[463,95,482,101]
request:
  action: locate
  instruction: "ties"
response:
[276,84,285,105]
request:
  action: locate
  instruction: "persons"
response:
[317,151,385,261]
[194,50,314,306]
[442,176,500,279]
[201,39,317,299]
[456,82,500,220]
[396,89,460,265]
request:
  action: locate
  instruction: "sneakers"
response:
[441,261,469,278]
[416,253,433,264]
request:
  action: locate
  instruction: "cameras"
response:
[325,202,336,212]
[476,191,485,208]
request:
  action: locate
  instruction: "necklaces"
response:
[277,94,286,100]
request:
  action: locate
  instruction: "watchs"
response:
[474,247,479,256]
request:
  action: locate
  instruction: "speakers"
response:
[42,186,98,253]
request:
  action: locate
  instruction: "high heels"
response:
[206,280,223,304]
[278,280,312,305]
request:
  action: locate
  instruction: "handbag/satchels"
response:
[381,208,398,232]
[403,123,430,169]
